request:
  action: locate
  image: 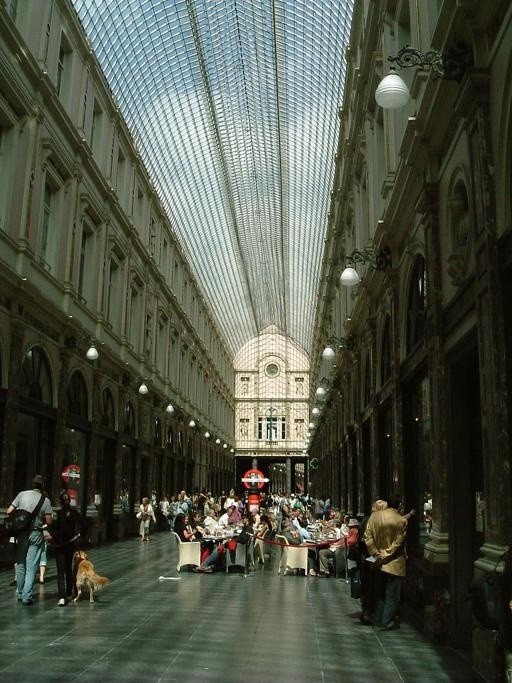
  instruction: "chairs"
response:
[171,527,358,576]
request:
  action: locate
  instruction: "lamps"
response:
[322,335,355,359]
[79,328,102,361]
[339,248,393,287]
[374,46,462,110]
[134,376,153,395]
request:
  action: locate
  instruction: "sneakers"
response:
[192,566,213,573]
[307,570,331,578]
[8,578,72,607]
[360,613,401,631]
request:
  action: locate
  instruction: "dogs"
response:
[71,550,110,603]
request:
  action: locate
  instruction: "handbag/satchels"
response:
[4,509,33,538]
[348,543,361,561]
[349,567,364,599]
